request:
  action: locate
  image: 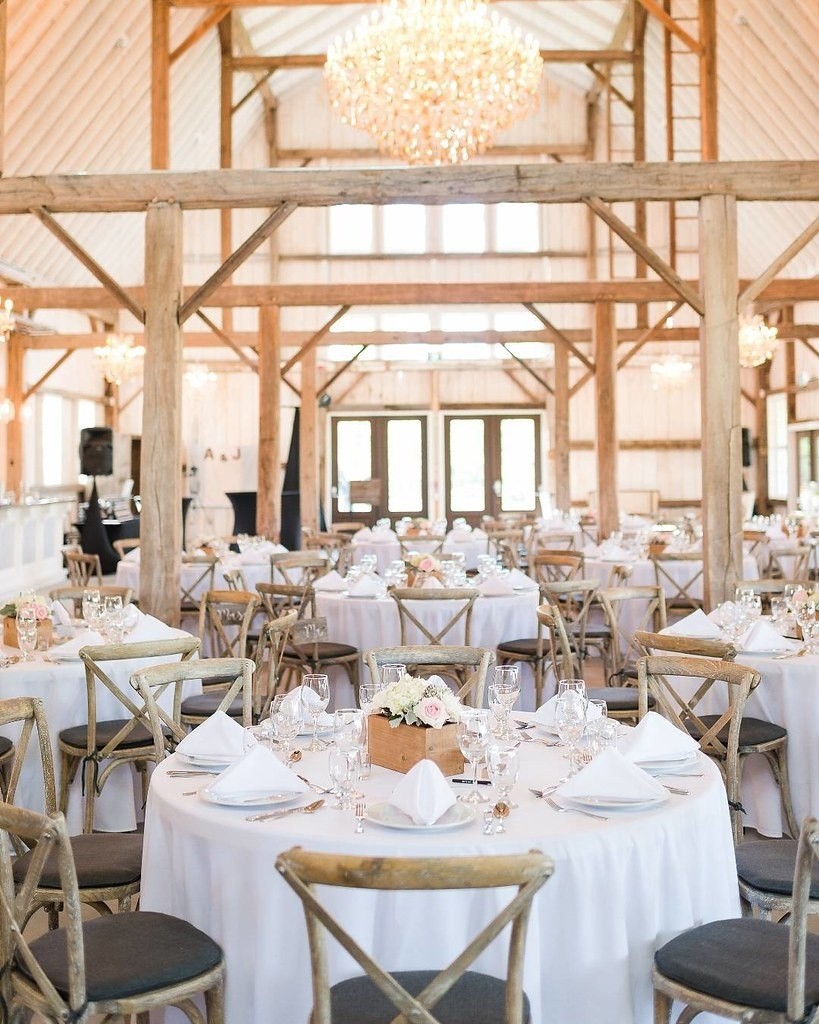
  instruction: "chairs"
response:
[59,637,201,835]
[650,813,818,1024]
[626,629,802,842]
[737,840,818,923]
[0,476,819,805]
[637,656,762,845]
[270,845,557,1024]
[1,695,144,934]
[1,800,228,1024]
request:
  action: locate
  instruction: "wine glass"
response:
[245,693,305,765]
[485,746,521,808]
[300,674,330,751]
[346,556,408,596]
[555,679,621,783]
[442,552,502,591]
[487,665,522,741]
[718,583,819,656]
[15,608,37,665]
[328,710,371,809]
[456,712,491,804]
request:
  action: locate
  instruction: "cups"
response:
[82,589,139,644]
[359,663,407,716]
[209,533,267,559]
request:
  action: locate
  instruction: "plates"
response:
[634,754,703,770]
[174,751,234,766]
[531,720,620,736]
[343,592,380,597]
[196,784,308,806]
[362,801,477,833]
[313,586,345,591]
[552,790,672,809]
[261,716,330,737]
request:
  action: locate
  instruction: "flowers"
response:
[791,588,818,611]
[0,588,52,619]
[403,551,440,573]
[365,673,469,727]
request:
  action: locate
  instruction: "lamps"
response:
[321,0,542,165]
[737,312,780,367]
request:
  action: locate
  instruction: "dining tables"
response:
[741,521,819,570]
[523,522,600,602]
[2,624,201,838]
[114,551,304,659]
[350,531,498,575]
[648,615,819,837]
[136,710,741,1022]
[575,546,759,658]
[304,578,540,715]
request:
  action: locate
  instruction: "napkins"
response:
[52,630,104,658]
[562,745,672,803]
[533,694,603,727]
[478,575,515,596]
[738,618,795,652]
[51,599,70,626]
[423,577,445,590]
[125,614,178,644]
[617,710,701,763]
[118,602,145,625]
[388,759,456,826]
[311,568,347,592]
[509,566,541,590]
[277,684,340,726]
[205,743,307,798]
[668,608,722,639]
[174,710,254,761]
[348,575,382,598]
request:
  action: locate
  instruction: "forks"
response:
[528,784,556,795]
[545,797,610,823]
[355,803,368,833]
[514,718,533,726]
[521,730,554,747]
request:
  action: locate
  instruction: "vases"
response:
[3,616,53,650]
[783,611,819,639]
[405,568,443,588]
[367,714,470,778]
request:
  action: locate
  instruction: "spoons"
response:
[246,800,326,822]
